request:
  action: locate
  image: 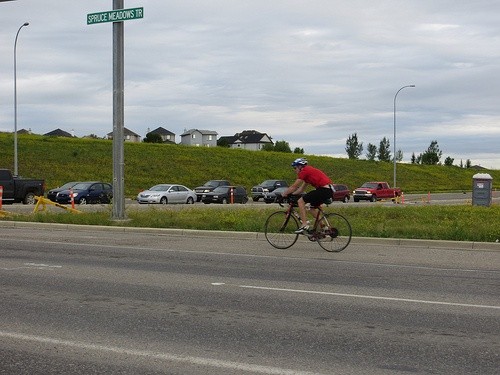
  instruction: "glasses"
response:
[294,167,297,170]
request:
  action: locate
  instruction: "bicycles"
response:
[264,193,352,252]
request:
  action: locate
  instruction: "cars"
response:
[136,184,197,204]
[331,184,350,203]
[264,187,307,204]
[46,181,113,205]
[201,186,248,204]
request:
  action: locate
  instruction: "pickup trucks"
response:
[0,169,44,205]
[352,181,400,202]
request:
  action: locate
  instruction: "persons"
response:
[280,157,336,233]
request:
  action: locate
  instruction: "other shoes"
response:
[295,225,309,232]
[319,225,328,234]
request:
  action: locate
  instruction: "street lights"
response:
[12,22,30,176]
[393,84,416,197]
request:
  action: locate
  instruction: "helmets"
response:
[291,157,308,166]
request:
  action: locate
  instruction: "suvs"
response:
[250,179,288,202]
[193,179,233,202]
